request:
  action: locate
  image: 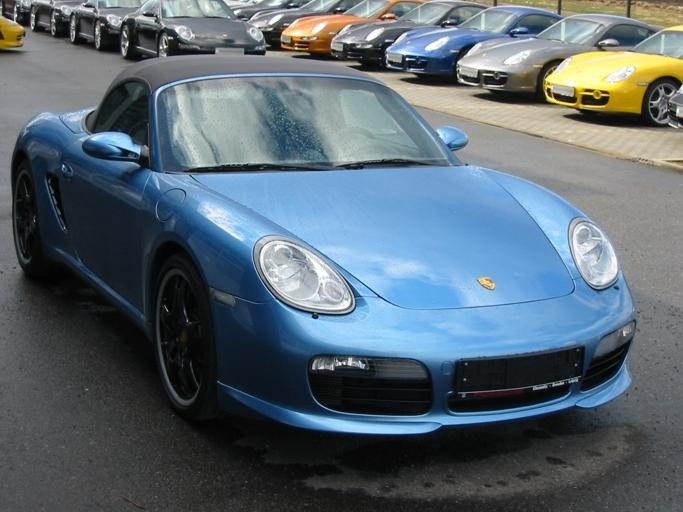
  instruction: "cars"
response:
[68,0,148,52]
[667,84,683,132]
[542,25,683,128]
[0,0,90,49]
[119,1,266,59]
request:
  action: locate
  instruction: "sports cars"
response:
[224,0,490,72]
[10,54,637,436]
[383,5,564,87]
[455,14,661,106]
